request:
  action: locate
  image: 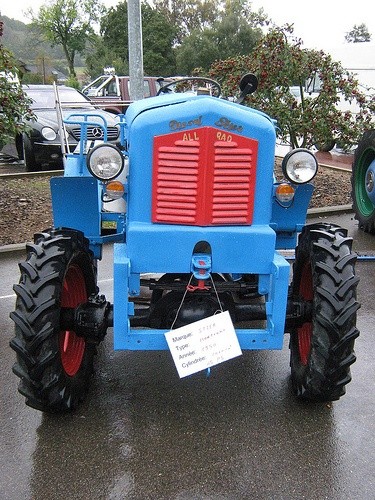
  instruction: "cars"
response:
[10,84,128,170]
[273,84,304,105]
[304,69,375,151]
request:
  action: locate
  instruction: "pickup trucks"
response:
[82,74,188,114]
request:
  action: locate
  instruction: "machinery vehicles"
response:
[8,73,363,416]
[351,129,375,233]
